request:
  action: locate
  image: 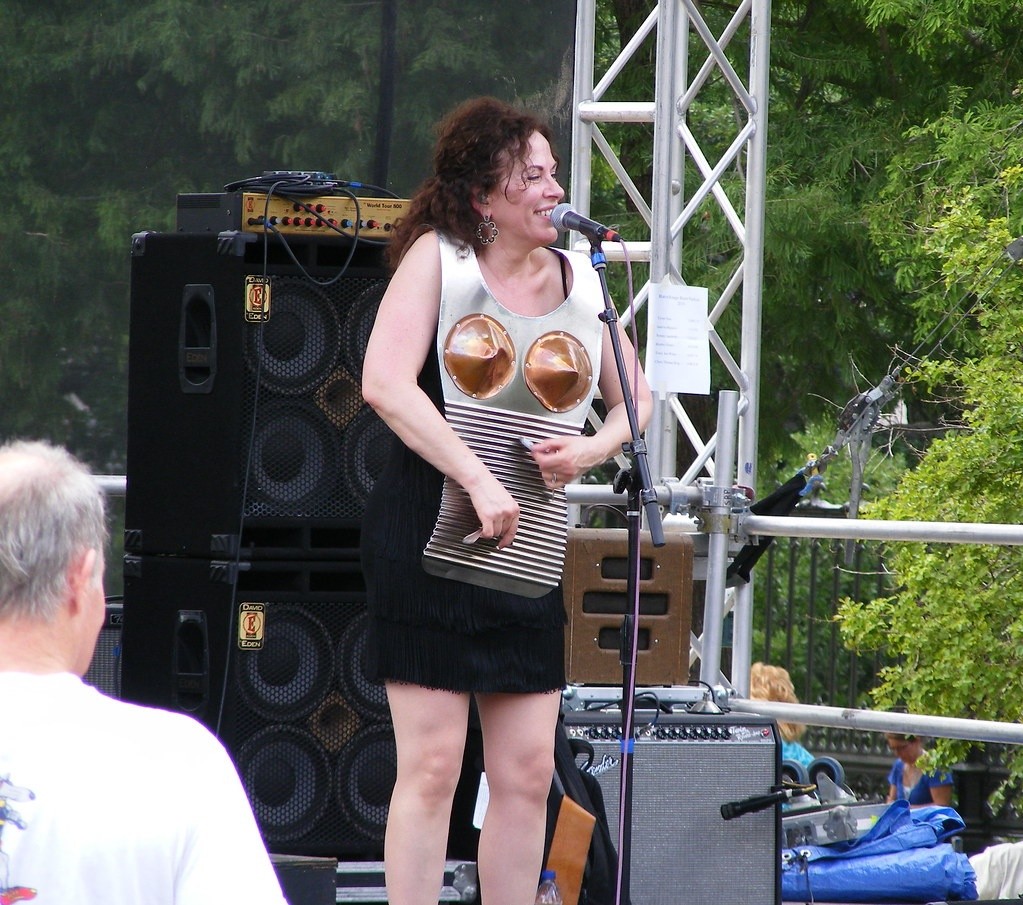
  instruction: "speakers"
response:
[119,231,460,863]
[559,712,785,905]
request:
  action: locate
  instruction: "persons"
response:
[2,440,291,905]
[883,732,953,812]
[751,661,816,770]
[356,97,658,904]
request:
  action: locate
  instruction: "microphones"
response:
[552,202,623,243]
[720,786,793,821]
